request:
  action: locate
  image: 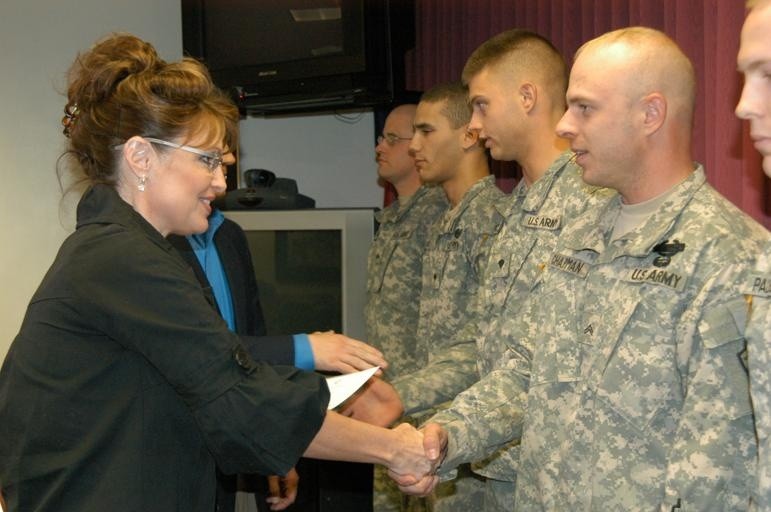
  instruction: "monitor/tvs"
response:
[220,210,376,344]
[180,0,415,116]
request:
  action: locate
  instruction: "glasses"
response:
[377,133,412,145]
[114,137,222,170]
